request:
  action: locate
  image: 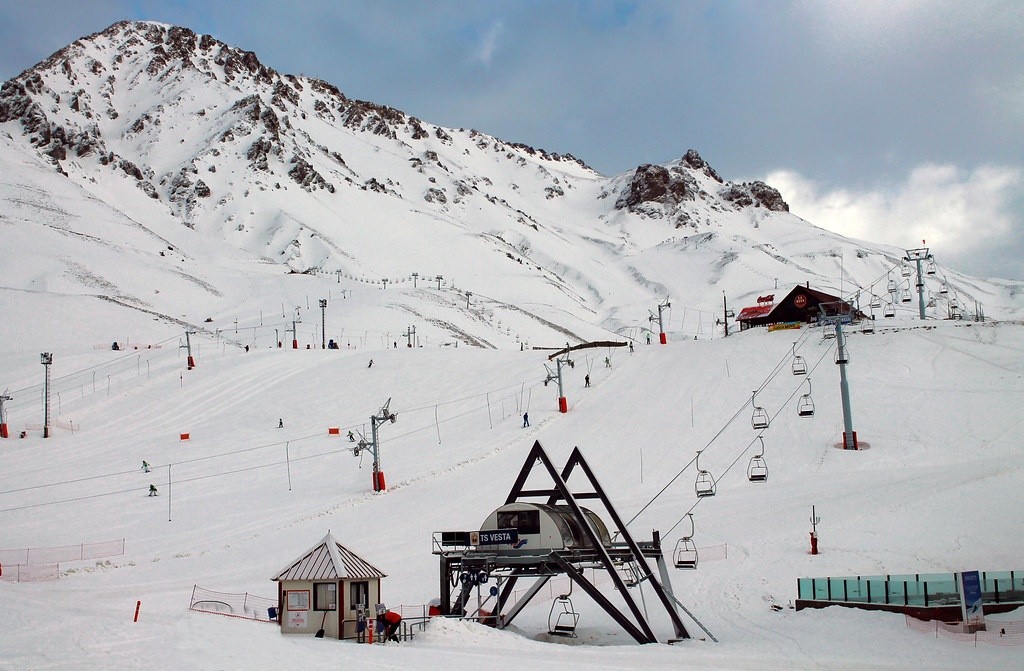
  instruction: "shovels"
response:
[314,611,326,638]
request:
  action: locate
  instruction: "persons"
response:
[329,339,334,349]
[604,357,611,367]
[112,342,120,350]
[647,333,650,344]
[279,418,283,428]
[394,341,397,348]
[347,431,354,442]
[368,359,374,368]
[244,345,249,352]
[279,341,282,348]
[375,611,402,644]
[521,342,523,351]
[140,460,158,497]
[630,341,634,352]
[524,412,529,427]
[585,374,590,387]
